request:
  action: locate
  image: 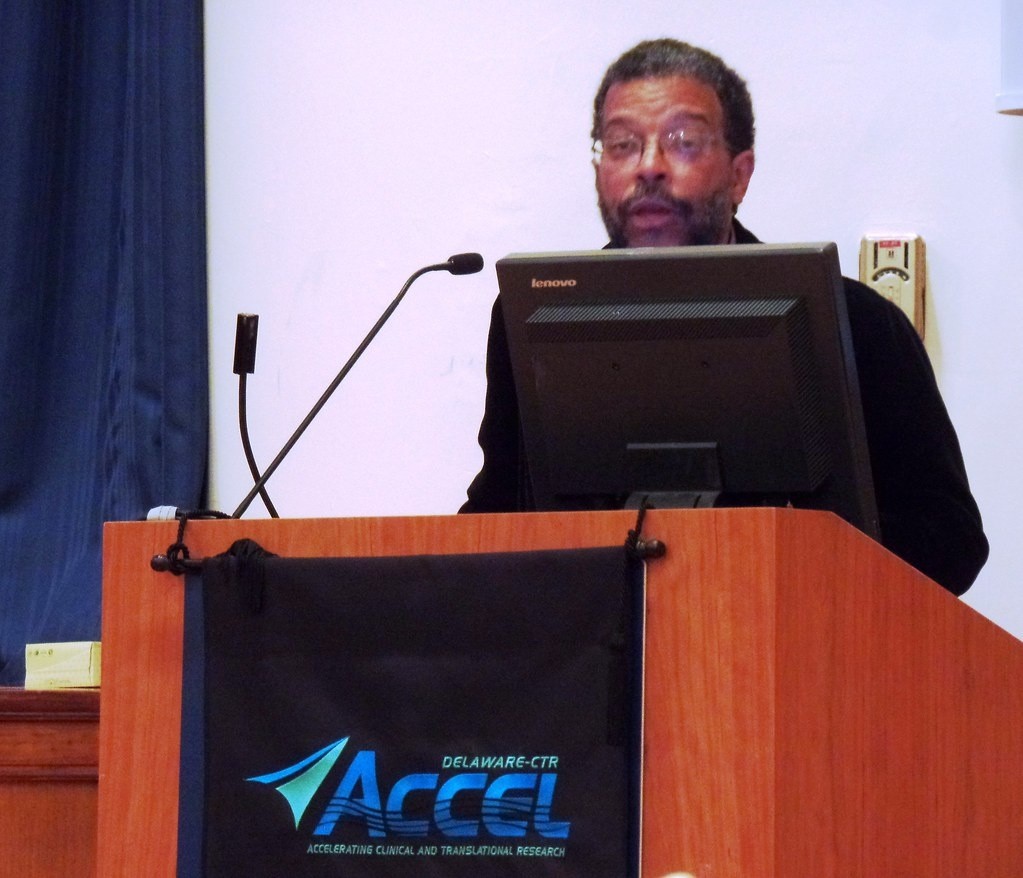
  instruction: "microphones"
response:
[230,253,485,519]
[233,313,280,519]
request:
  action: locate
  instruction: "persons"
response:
[456,40,987,602]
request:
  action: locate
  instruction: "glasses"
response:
[591,128,737,164]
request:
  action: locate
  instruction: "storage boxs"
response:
[23,642,103,688]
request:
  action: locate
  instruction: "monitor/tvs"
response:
[495,240,882,546]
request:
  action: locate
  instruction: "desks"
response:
[0,686,98,878]
